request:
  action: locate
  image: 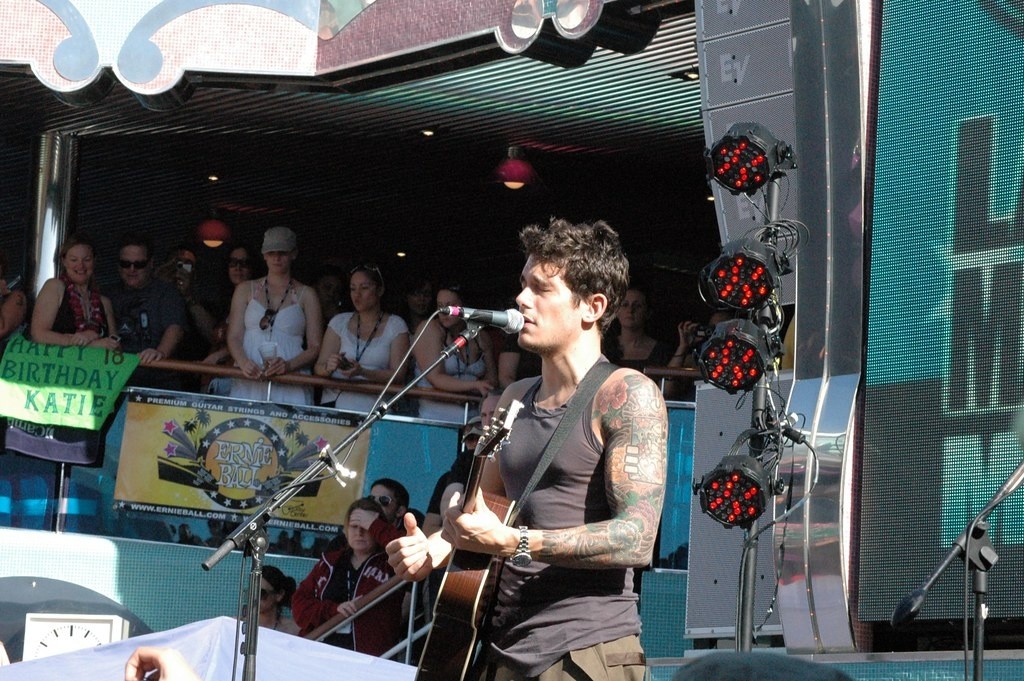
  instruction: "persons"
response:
[605,284,735,402]
[0,227,549,425]
[385,214,669,680]
[254,394,503,658]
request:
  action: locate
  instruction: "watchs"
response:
[510,526,532,568]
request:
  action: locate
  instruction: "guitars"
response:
[414,399,524,681]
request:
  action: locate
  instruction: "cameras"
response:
[173,258,192,277]
[336,354,350,371]
[693,324,716,336]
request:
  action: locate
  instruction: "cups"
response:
[258,344,277,368]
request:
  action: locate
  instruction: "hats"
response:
[261,227,297,254]
[460,417,483,444]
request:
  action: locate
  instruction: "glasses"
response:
[368,495,398,507]
[118,260,149,269]
[350,262,383,278]
[267,249,295,259]
[228,259,251,268]
[261,588,278,600]
[462,421,483,433]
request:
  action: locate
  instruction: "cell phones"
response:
[5,274,22,288]
[109,334,122,341]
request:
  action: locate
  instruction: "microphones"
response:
[438,305,525,334]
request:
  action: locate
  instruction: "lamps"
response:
[700,456,769,526]
[704,122,785,196]
[492,147,534,189]
[196,213,233,247]
[702,237,779,310]
[698,320,769,394]
